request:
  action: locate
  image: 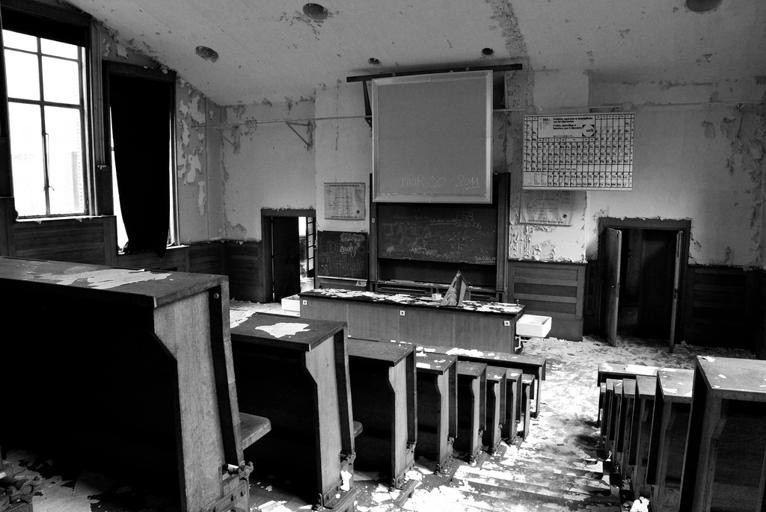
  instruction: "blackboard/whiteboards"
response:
[378,175,498,266]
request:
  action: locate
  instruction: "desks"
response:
[593,348,765,512]
[0,252,549,512]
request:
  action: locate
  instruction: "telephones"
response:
[317,231,368,282]
